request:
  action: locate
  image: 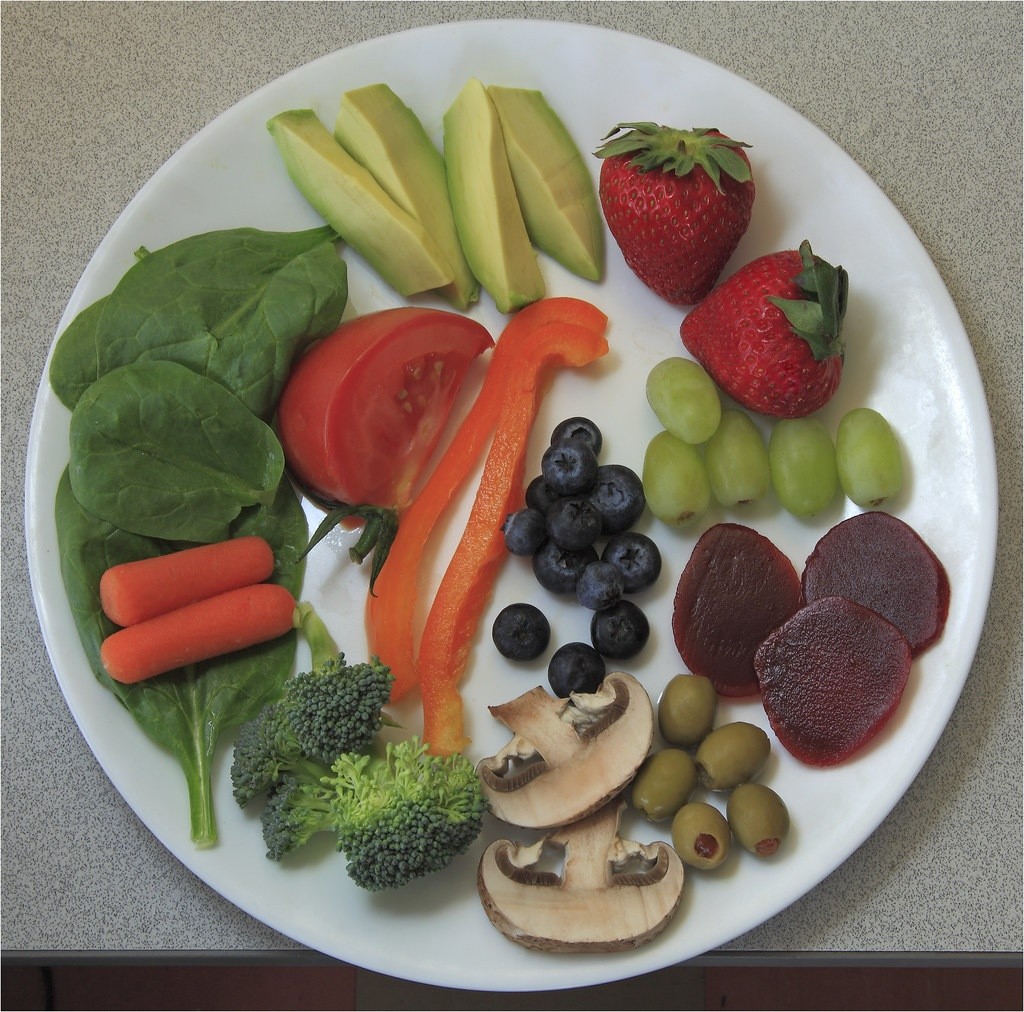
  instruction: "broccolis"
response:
[230,652,488,893]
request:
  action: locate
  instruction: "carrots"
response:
[100,584,296,683]
[98,536,275,628]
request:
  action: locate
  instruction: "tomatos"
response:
[276,307,496,514]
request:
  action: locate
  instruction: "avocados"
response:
[265,78,605,314]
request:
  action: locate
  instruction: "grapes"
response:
[641,358,902,528]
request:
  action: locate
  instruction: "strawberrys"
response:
[678,240,849,417]
[591,122,756,306]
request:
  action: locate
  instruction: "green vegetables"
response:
[46,224,354,849]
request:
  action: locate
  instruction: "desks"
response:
[0,0,1024,975]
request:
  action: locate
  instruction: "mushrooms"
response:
[474,672,654,829]
[476,796,685,953]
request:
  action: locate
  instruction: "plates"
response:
[26,14,999,991]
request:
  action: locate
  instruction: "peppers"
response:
[365,295,610,756]
